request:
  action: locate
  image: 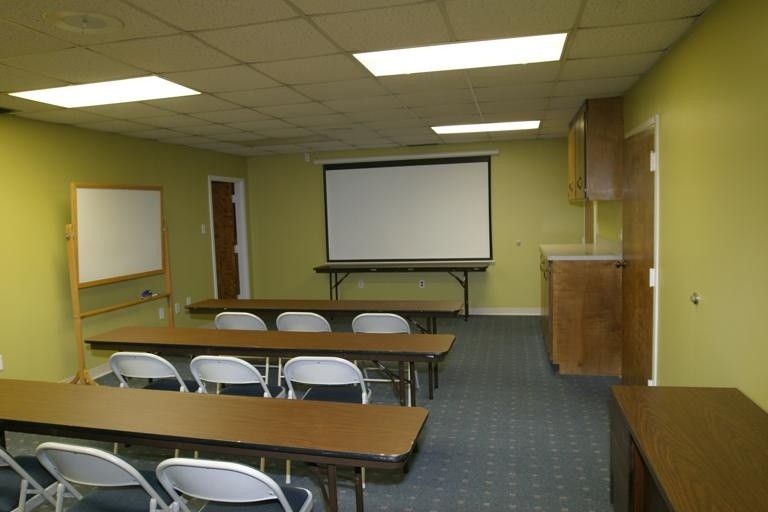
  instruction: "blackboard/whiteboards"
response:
[71,183,165,290]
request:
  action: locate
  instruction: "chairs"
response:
[188,355,286,472]
[351,314,420,408]
[1,451,82,511]
[276,314,333,385]
[214,314,286,395]
[282,357,374,490]
[110,352,206,459]
[35,442,192,510]
[156,457,314,510]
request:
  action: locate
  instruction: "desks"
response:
[607,384,768,512]
[184,298,465,400]
[313,262,488,322]
[84,326,456,474]
[0,378,431,510]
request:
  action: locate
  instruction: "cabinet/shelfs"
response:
[567,95,625,201]
[538,242,623,379]
